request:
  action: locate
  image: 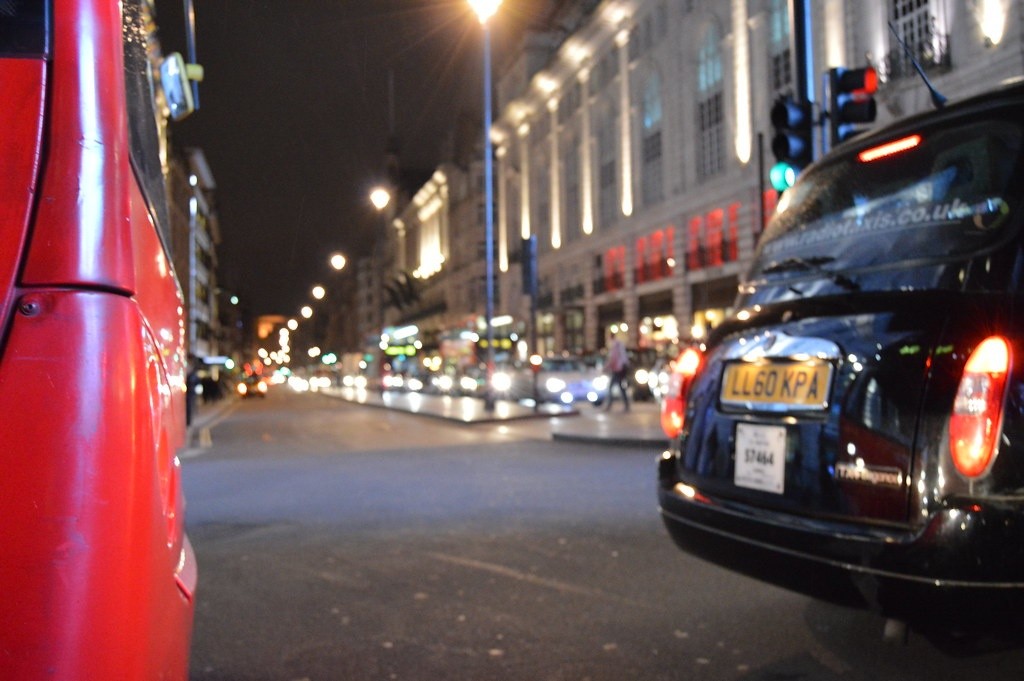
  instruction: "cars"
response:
[238,376,269,400]
[655,77,1024,654]
[281,329,672,425]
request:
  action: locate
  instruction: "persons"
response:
[600,330,631,413]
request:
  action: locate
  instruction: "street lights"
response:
[465,1,505,406]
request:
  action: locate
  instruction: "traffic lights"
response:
[823,66,880,155]
[767,96,821,198]
